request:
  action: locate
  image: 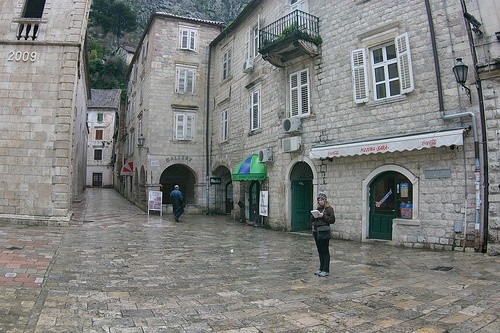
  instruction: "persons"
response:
[170,184,185,222]
[310,192,336,277]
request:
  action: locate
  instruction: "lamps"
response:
[464,12,482,36]
[452,58,472,102]
[136,134,149,153]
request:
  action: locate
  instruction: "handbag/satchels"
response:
[317,225,332,239]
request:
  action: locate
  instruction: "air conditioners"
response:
[258,148,273,162]
[242,59,253,73]
[282,116,301,133]
[282,136,301,153]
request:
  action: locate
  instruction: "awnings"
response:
[232,154,266,181]
[119,162,133,176]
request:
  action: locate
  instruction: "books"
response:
[311,210,320,218]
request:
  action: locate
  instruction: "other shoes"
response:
[314,270,322,275]
[320,271,330,276]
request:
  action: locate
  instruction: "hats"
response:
[317,192,327,200]
